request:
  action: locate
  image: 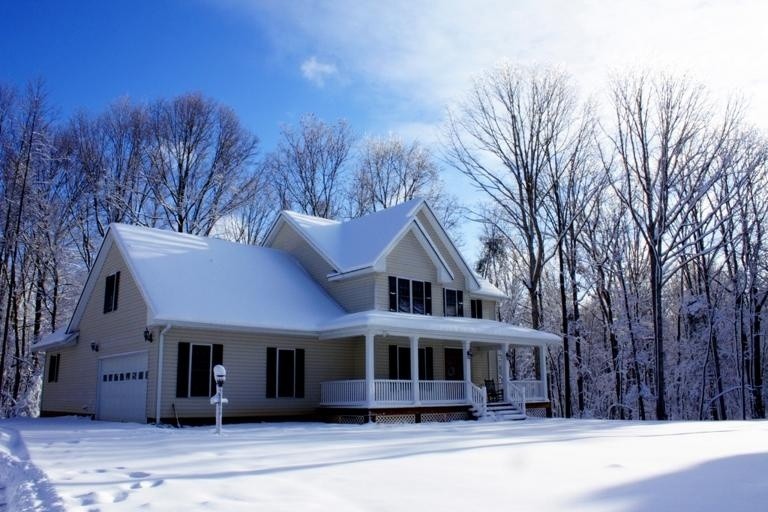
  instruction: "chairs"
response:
[484,378,504,403]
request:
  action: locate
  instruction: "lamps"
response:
[90,342,98,352]
[143,327,152,343]
